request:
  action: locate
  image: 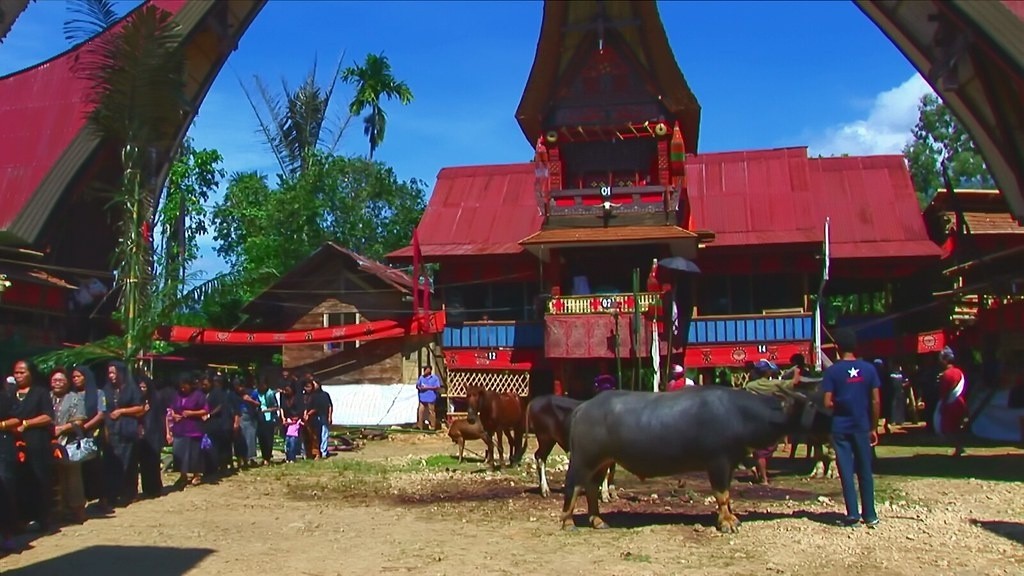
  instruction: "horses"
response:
[448,384,528,472]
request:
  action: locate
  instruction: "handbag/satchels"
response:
[200,432,216,452]
[43,435,69,472]
[64,430,99,465]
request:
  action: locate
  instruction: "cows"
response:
[522,386,830,533]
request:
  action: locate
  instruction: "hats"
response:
[753,358,779,374]
[669,363,685,375]
[421,365,432,370]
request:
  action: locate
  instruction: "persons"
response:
[413,365,442,431]
[0,358,334,550]
[933,345,972,457]
[665,363,695,392]
[744,353,811,486]
[823,326,883,526]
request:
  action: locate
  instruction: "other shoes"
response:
[172,457,270,490]
[867,516,880,525]
[848,517,860,525]
[951,448,966,458]
